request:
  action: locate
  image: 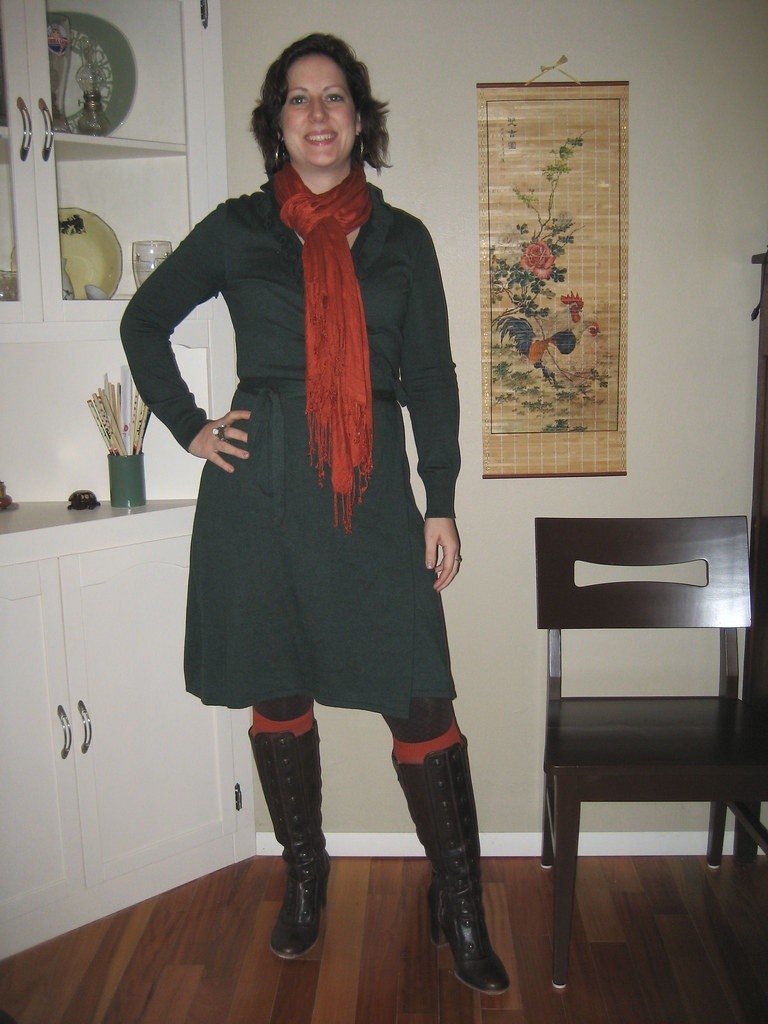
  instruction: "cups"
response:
[47,14,72,133]
[107,453,146,508]
[132,241,172,290]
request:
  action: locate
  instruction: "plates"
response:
[11,208,122,299]
[46,11,135,138]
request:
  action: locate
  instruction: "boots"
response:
[391,732,509,996]
[247,719,329,959]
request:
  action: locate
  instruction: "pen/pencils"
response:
[86,364,152,457]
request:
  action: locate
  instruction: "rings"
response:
[456,555,462,562]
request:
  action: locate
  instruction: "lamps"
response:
[76,36,112,138]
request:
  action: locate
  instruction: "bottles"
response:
[61,259,74,300]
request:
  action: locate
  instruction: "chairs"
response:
[534,515,767,989]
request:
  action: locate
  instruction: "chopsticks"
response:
[87,365,151,456]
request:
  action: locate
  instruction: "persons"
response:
[121,35,509,994]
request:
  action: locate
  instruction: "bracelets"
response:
[213,424,226,439]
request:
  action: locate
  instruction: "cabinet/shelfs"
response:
[1,1,210,325]
[0,503,237,962]
